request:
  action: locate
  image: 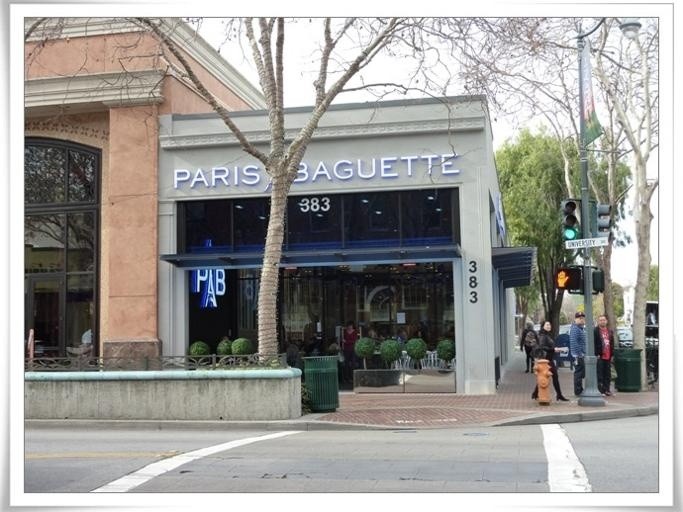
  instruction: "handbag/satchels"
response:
[529,346,546,360]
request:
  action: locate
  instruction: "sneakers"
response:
[601,390,616,397]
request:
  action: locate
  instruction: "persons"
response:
[530,321,568,401]
[593,315,616,395]
[520,323,538,373]
[341,321,358,383]
[417,321,424,338]
[568,310,588,396]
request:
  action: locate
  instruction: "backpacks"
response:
[524,330,537,347]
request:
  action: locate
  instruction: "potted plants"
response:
[353,336,455,394]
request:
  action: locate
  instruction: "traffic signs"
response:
[565,238,610,250]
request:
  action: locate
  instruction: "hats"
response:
[575,311,586,317]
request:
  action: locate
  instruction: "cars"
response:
[615,330,635,350]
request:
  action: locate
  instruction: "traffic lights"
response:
[594,204,613,244]
[561,200,583,243]
[556,266,582,290]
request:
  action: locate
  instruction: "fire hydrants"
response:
[532,359,552,406]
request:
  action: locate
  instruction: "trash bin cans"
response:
[612,348,643,392]
[301,356,339,412]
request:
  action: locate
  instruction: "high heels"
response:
[556,394,569,402]
[532,389,539,401]
